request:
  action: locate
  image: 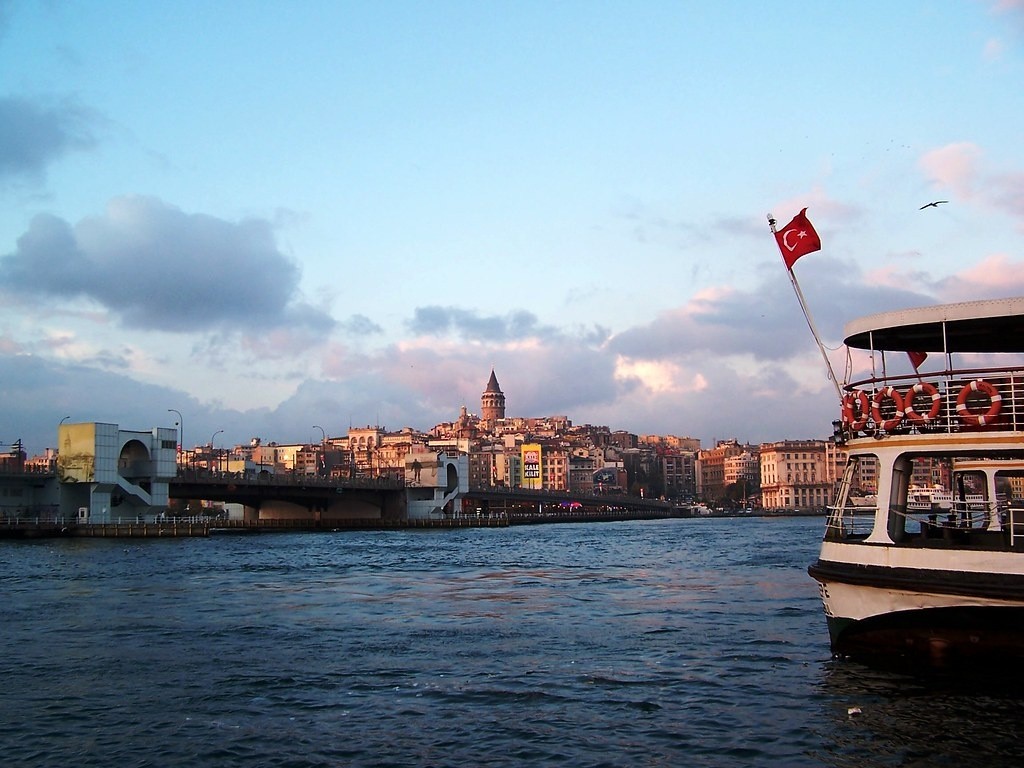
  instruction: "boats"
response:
[806,297,1024,672]
[928,491,984,513]
[828,481,950,515]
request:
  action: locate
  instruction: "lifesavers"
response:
[871,386,905,428]
[846,389,869,430]
[955,381,1003,426]
[841,392,849,430]
[904,383,941,424]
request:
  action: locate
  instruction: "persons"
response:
[411,459,423,482]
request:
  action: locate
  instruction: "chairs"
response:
[918,514,991,543]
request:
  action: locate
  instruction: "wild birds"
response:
[918,201,948,210]
[331,528,340,532]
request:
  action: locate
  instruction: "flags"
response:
[774,207,820,271]
[908,351,927,369]
